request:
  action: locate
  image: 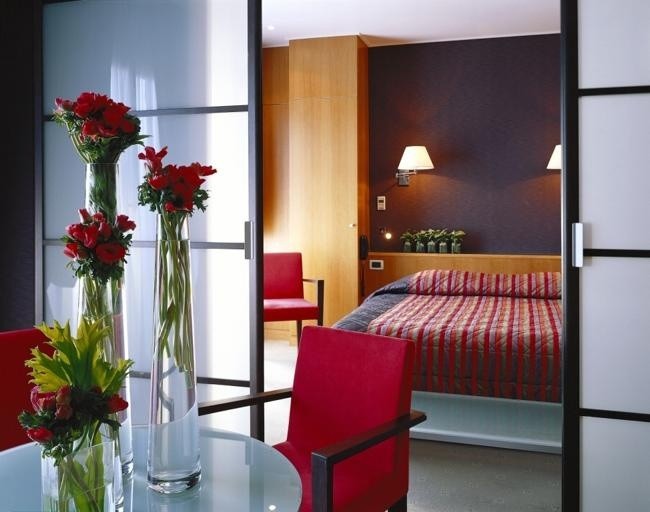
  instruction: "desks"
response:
[0,424,303,512]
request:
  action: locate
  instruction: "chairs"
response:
[0,327,57,451]
[197,325,426,512]
[261,251,324,351]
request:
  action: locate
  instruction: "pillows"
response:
[362,270,562,303]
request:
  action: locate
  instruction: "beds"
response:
[328,292,563,456]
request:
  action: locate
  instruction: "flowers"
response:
[137,146,217,390]
[52,91,152,316]
[61,208,136,457]
[18,312,135,512]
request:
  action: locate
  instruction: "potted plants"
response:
[398,229,467,253]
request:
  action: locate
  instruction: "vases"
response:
[145,214,202,496]
[41,434,116,512]
[73,275,125,511]
[75,163,134,476]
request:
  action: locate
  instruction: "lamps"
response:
[395,145,434,186]
[546,144,562,170]
